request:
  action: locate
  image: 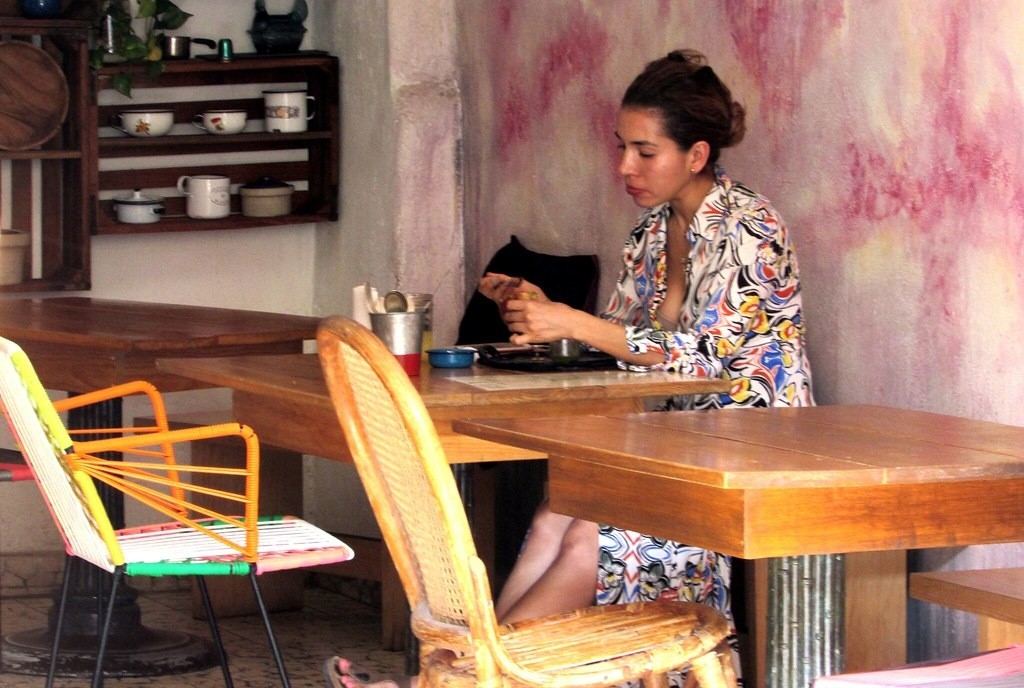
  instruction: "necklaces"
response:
[676,251,687,269]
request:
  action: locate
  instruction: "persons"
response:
[323,49,818,688]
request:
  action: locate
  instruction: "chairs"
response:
[316,312,736,687]
[0,336,357,688]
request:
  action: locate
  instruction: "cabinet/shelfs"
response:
[0,17,94,295]
[87,53,340,235]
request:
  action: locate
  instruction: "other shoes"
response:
[325,656,411,688]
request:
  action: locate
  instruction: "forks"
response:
[498,278,523,287]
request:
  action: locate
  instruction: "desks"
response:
[1,290,324,677]
[148,345,733,684]
[446,404,1024,688]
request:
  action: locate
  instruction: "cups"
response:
[261,89,316,134]
[176,174,231,220]
[369,311,425,377]
[192,109,247,135]
[111,109,175,138]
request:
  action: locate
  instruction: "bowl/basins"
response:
[426,348,475,369]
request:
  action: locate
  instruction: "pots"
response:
[113,188,166,224]
[155,35,217,60]
[238,173,295,219]
